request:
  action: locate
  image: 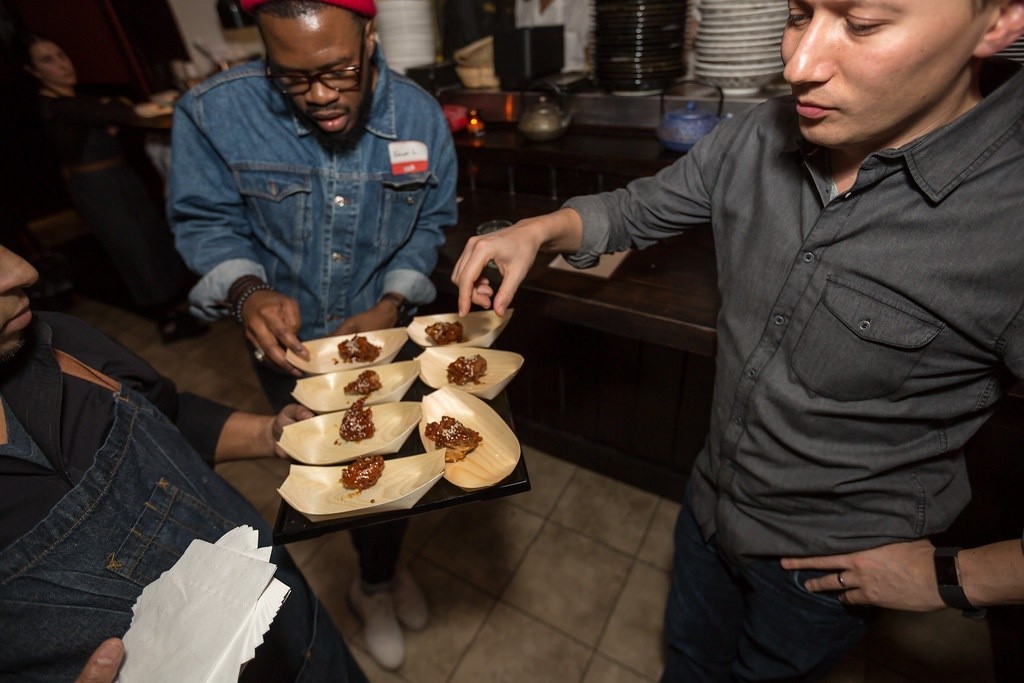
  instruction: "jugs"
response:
[655,79,724,153]
[515,77,576,143]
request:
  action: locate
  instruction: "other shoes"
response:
[389,568,427,628]
[158,307,212,344]
[350,575,404,670]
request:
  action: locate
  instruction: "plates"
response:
[990,34,1024,62]
[586,0,691,96]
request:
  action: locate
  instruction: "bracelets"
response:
[386,295,401,308]
[237,284,277,323]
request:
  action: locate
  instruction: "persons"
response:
[167,1,492,670]
[11,31,210,344]
[0,239,371,683]
[449,0,1024,683]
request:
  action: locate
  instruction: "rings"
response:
[838,572,846,587]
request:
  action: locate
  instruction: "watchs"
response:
[933,547,972,610]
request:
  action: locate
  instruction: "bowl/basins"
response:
[275,307,525,522]
[692,1,792,96]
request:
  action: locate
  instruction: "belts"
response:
[64,156,126,174]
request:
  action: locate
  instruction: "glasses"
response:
[264,26,364,97]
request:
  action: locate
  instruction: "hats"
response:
[241,0,377,16]
